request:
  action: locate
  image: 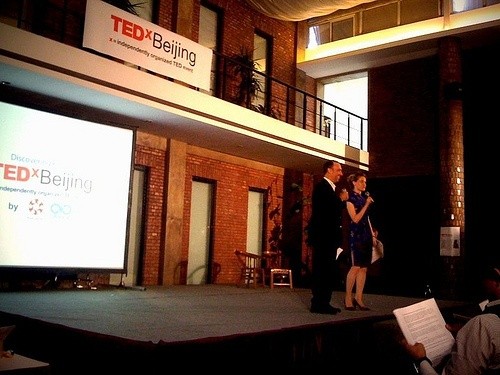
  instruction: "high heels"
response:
[352,299,370,310]
[343,297,356,311]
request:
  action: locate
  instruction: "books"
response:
[393,297,456,362]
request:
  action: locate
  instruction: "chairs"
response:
[234,249,266,289]
[263,251,293,289]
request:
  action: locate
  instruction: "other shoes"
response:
[310,301,341,315]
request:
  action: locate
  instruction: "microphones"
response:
[342,188,347,192]
[364,191,370,198]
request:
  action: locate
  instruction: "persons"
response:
[309,160,349,315]
[343,171,379,310]
[364,226,386,295]
[395,225,500,375]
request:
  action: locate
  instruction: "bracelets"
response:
[414,356,433,373]
[372,236,376,238]
